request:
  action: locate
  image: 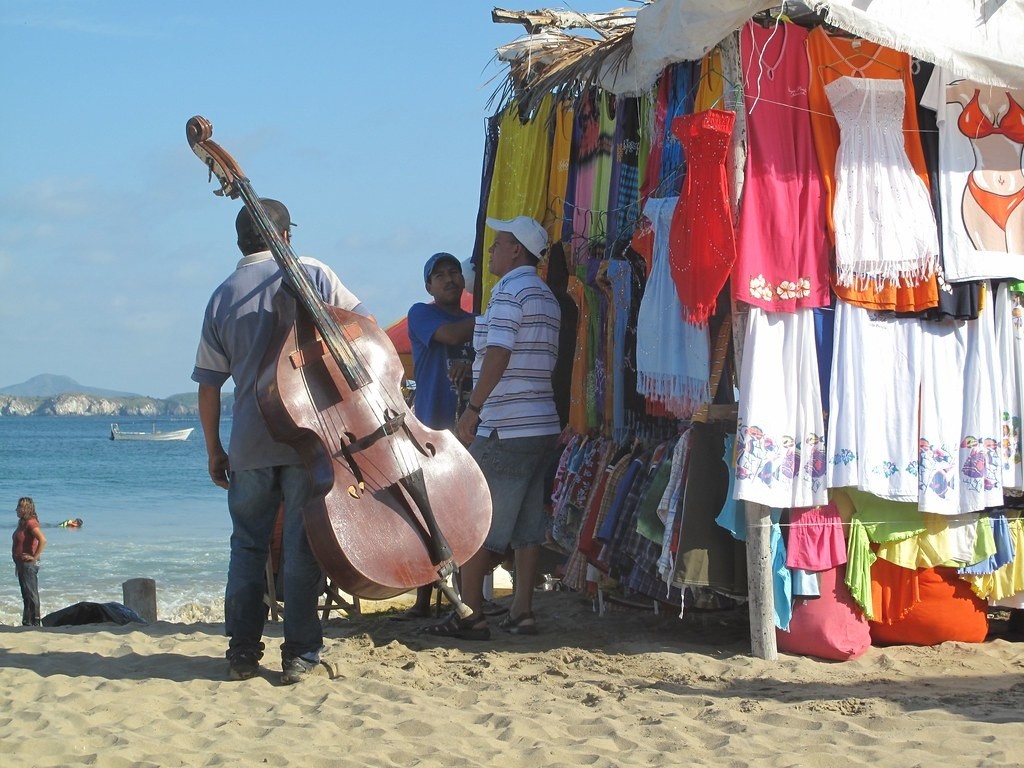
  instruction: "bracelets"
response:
[466,402,480,411]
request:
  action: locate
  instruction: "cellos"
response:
[184,113,494,621]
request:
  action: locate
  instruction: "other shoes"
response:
[405,603,431,618]
[482,598,509,615]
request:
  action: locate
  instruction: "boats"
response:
[110,423,193,442]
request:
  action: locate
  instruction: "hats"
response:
[423,253,463,284]
[236,198,297,246]
[485,214,549,261]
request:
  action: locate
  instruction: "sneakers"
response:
[227,652,260,680]
[281,651,346,684]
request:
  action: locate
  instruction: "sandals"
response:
[499,610,538,636]
[417,610,491,640]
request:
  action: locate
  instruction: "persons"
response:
[191,198,377,685]
[407,253,476,617]
[66,519,83,527]
[12,497,47,626]
[419,215,564,642]
